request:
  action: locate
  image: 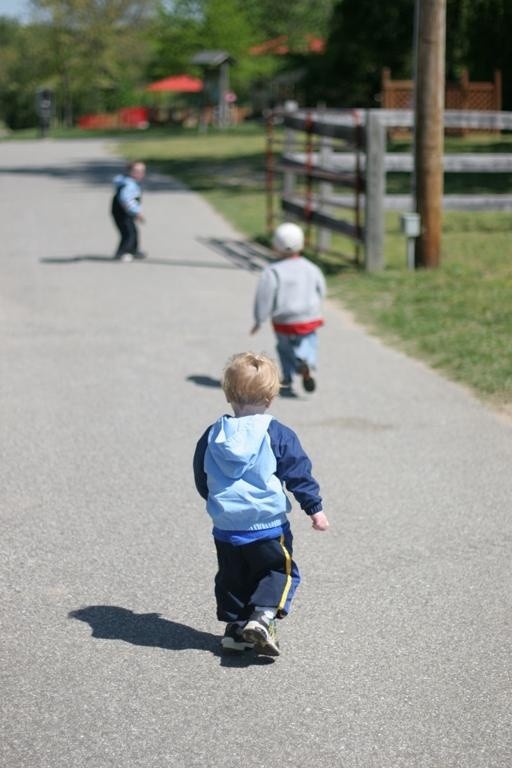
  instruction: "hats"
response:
[272,223,305,254]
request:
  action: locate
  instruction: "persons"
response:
[250,220,327,394]
[191,350,331,657]
[36,87,53,137]
[110,161,150,262]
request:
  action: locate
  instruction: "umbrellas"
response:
[147,74,205,93]
[248,31,324,57]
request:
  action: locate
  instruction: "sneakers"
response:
[298,362,314,392]
[221,624,255,651]
[242,610,280,657]
